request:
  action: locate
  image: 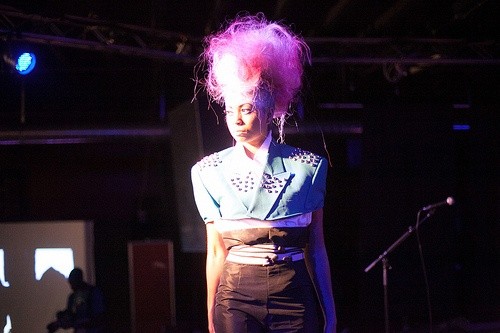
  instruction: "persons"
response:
[190,63,337,333]
[62,267,107,333]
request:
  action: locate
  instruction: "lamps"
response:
[0,40,36,75]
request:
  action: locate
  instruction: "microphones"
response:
[422,197,455,212]
